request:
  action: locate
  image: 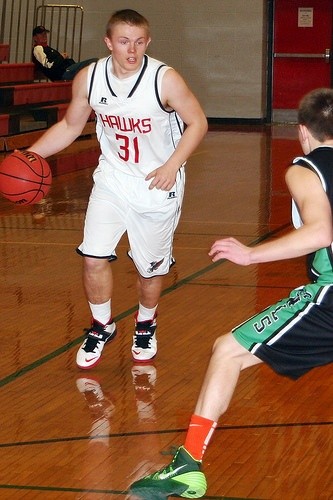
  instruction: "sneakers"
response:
[74,317,117,371]
[129,444,208,500]
[132,310,160,361]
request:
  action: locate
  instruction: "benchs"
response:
[0,44,101,177]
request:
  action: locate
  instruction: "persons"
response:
[13,9,209,368]
[32,25,101,81]
[69,363,165,500]
[126,88,333,500]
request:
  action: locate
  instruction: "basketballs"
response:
[0,150,52,208]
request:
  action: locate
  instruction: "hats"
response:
[32,25,51,35]
